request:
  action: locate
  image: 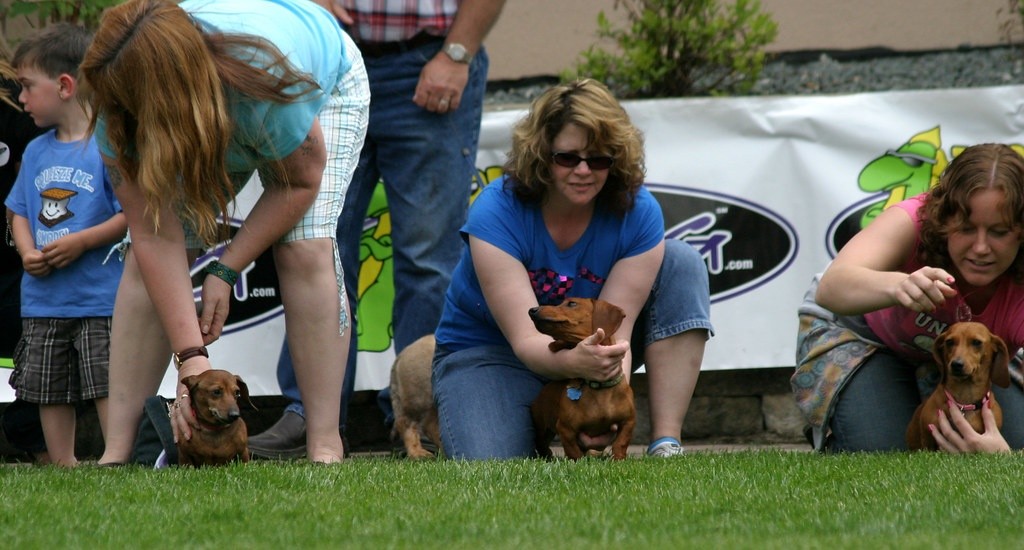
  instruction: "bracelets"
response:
[206,261,239,287]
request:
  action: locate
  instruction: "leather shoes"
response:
[397,437,439,458]
[248,410,349,459]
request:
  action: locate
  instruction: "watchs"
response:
[173,346,209,370]
[441,43,472,64]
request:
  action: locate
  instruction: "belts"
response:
[357,31,438,57]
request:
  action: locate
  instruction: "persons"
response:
[243,1,508,464]
[1,29,49,358]
[431,77,717,461]
[791,143,1024,455]
[4,22,127,466]
[75,0,372,467]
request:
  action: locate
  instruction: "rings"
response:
[182,394,189,399]
[440,99,449,104]
[168,412,173,417]
[174,402,181,408]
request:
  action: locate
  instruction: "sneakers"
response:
[646,437,684,456]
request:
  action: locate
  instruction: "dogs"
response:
[389,334,442,459]
[528,297,636,459]
[178,369,259,465]
[905,322,1010,451]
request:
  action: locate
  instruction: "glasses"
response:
[548,152,618,170]
